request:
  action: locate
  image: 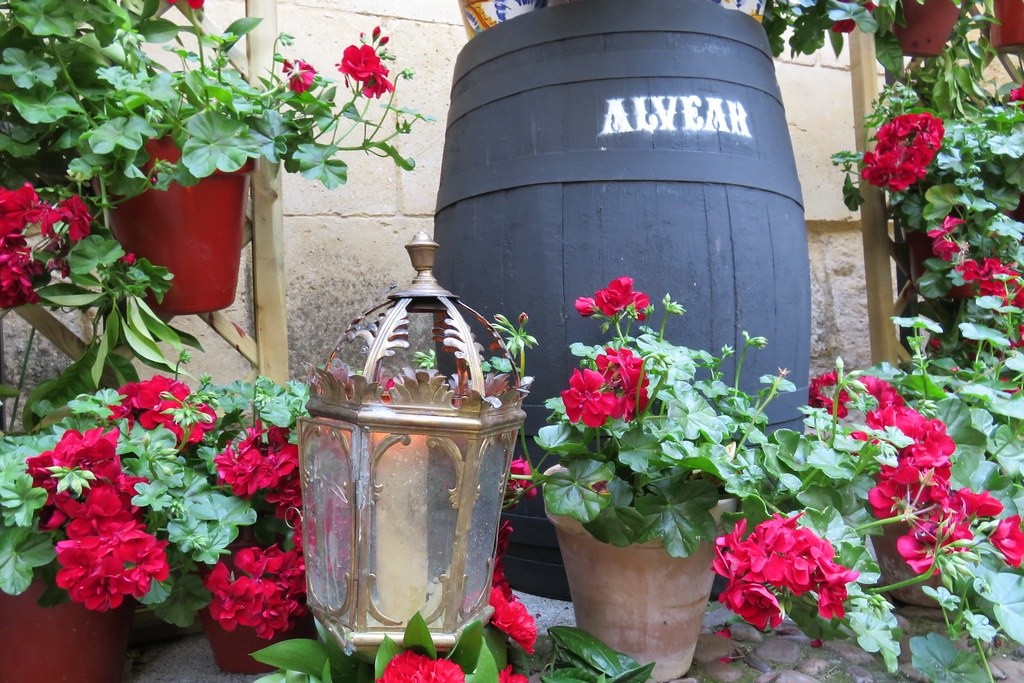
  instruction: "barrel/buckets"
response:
[432,1,810,600]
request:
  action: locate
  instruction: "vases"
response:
[0,575,125,683]
[544,491,738,683]
[867,506,953,608]
[0,0,1024,683]
[93,133,257,314]
[893,0,960,57]
[200,580,296,674]
[990,0,1024,48]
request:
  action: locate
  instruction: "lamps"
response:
[295,231,526,661]
